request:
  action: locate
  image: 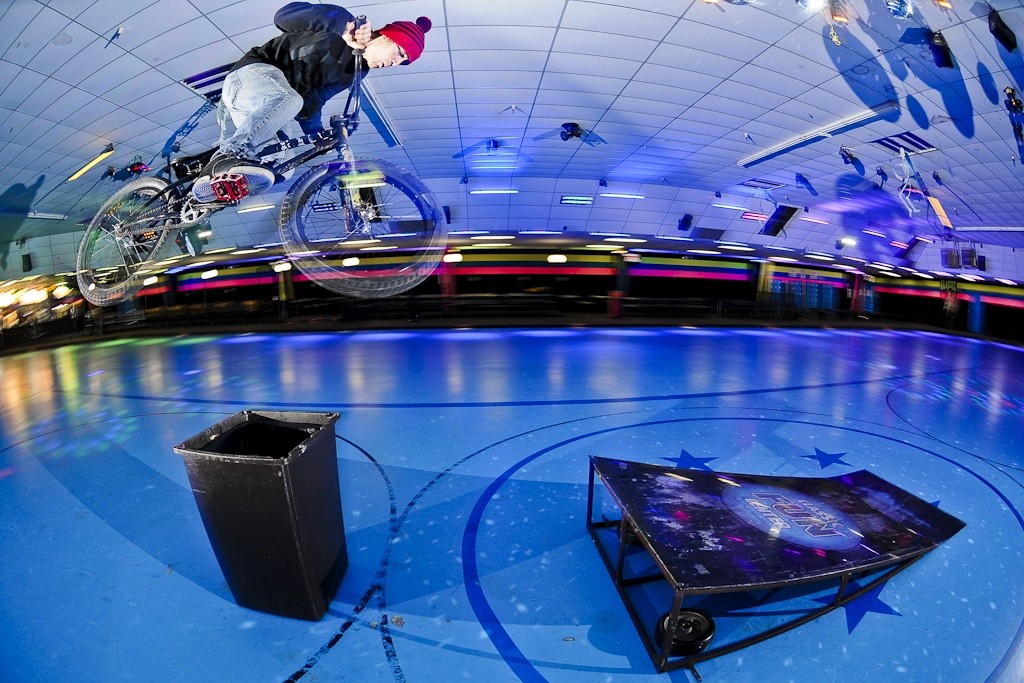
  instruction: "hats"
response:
[373,16,432,65]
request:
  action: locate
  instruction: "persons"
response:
[191,0,428,205]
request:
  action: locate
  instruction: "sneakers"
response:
[192,154,275,204]
[185,228,203,257]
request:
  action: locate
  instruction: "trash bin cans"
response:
[172,408,350,624]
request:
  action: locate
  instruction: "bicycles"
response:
[75,15,447,308]
